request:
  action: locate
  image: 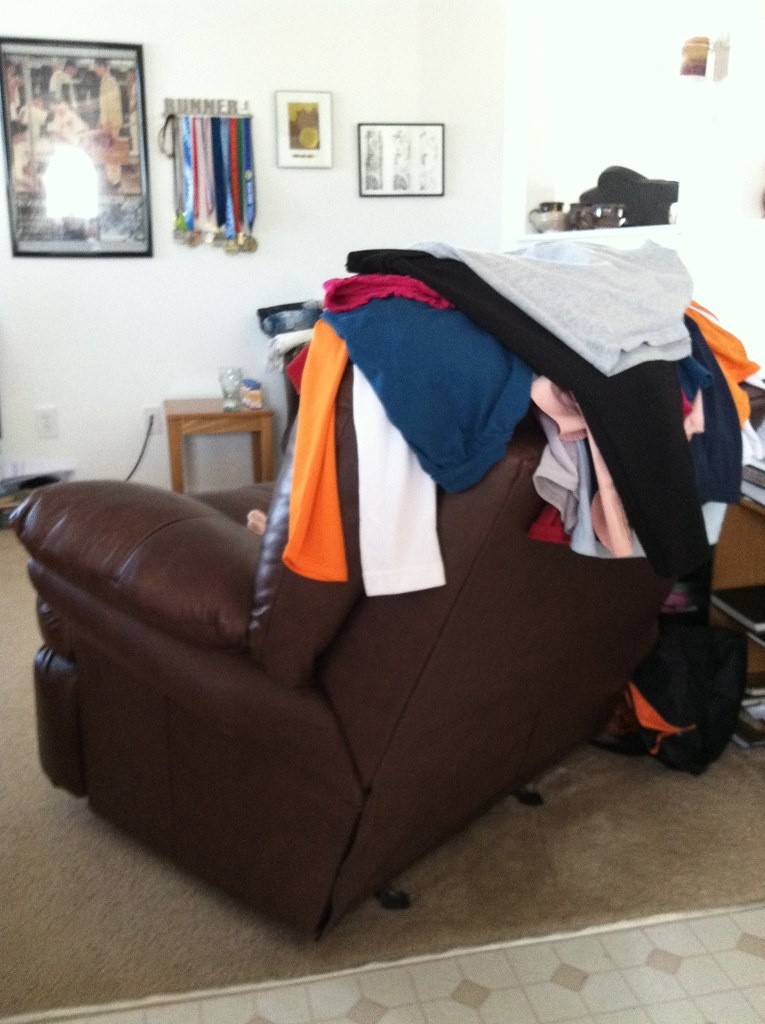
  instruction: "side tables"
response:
[710,494,765,736]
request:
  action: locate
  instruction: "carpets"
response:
[2,489,765,1023]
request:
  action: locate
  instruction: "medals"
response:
[173,228,257,256]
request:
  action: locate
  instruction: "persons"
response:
[4,57,142,190]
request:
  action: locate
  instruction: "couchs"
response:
[7,303,731,956]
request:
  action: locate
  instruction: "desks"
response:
[163,398,278,498]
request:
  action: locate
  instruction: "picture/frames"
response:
[361,122,447,196]
[274,91,334,169]
[0,37,151,252]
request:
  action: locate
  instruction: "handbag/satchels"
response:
[585,606,751,777]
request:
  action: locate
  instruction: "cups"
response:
[218,365,241,410]
[568,203,629,229]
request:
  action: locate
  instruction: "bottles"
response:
[241,379,263,410]
[529,201,570,233]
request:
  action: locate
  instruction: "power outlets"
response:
[142,404,164,436]
[33,406,63,440]
